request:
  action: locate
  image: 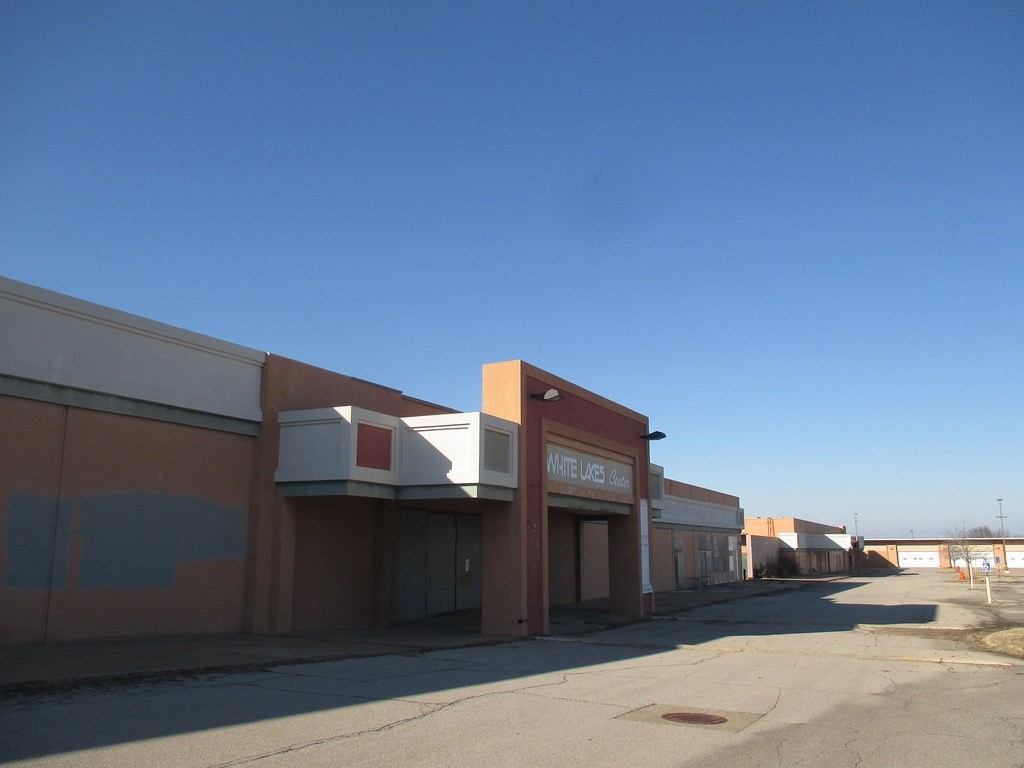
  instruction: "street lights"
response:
[996,498,1008,570]
[909,529,913,537]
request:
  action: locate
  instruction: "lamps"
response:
[529,388,566,403]
[640,431,666,440]
